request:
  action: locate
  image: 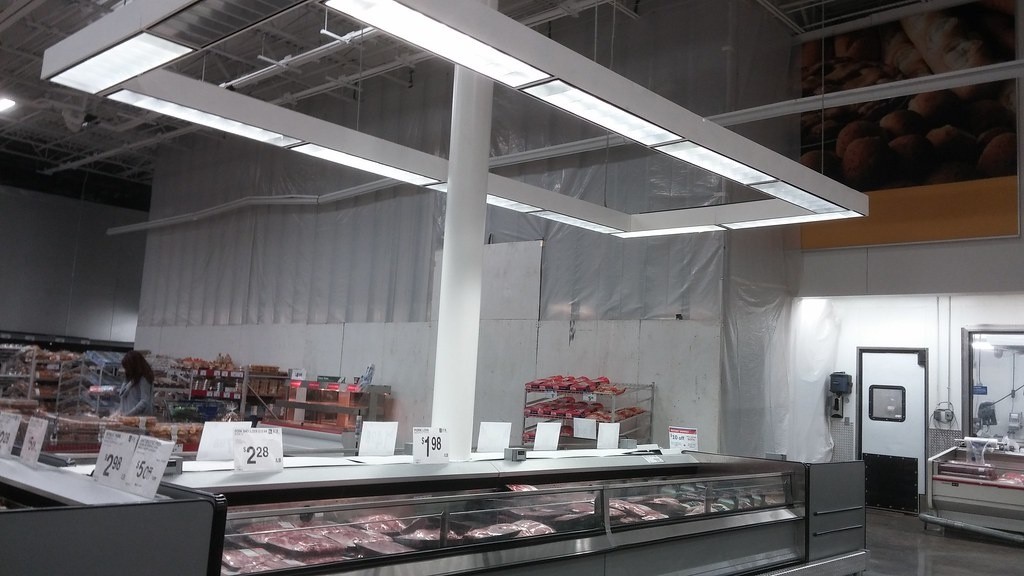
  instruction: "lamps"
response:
[41,0,868,240]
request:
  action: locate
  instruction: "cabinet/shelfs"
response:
[0,344,391,433]
[521,382,656,450]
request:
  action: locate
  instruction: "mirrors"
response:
[959,323,1024,455]
